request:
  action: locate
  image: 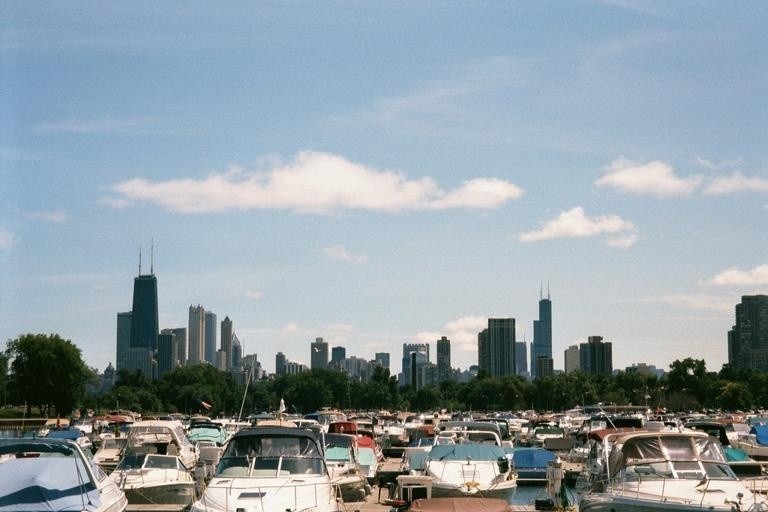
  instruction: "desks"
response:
[395,475,434,504]
[377,456,406,503]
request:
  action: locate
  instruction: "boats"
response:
[0,397,768,512]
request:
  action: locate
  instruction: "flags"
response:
[278,398,286,413]
[196,398,211,411]
